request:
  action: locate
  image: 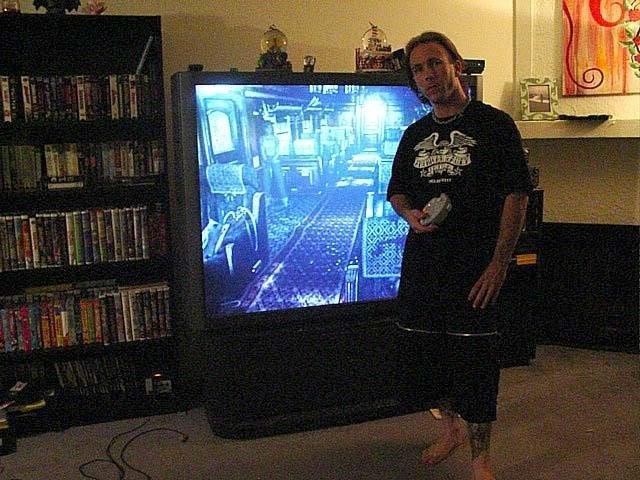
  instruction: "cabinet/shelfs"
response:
[496,187,544,366]
[0,11,181,435]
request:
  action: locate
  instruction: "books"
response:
[0,278,172,351]
[0,202,171,274]
[1,72,164,125]
[1,139,167,188]
[0,355,172,406]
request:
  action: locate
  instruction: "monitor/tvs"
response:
[172,72,483,333]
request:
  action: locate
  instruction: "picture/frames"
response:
[562,1,640,97]
[520,77,558,121]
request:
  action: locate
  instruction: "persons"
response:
[388,32,528,476]
